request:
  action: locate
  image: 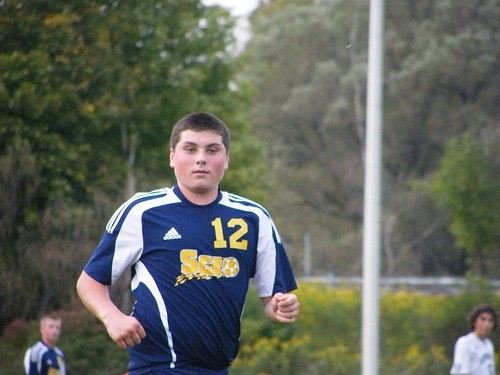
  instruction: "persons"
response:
[76,111,301,375]
[23,312,68,374]
[449,307,499,374]
